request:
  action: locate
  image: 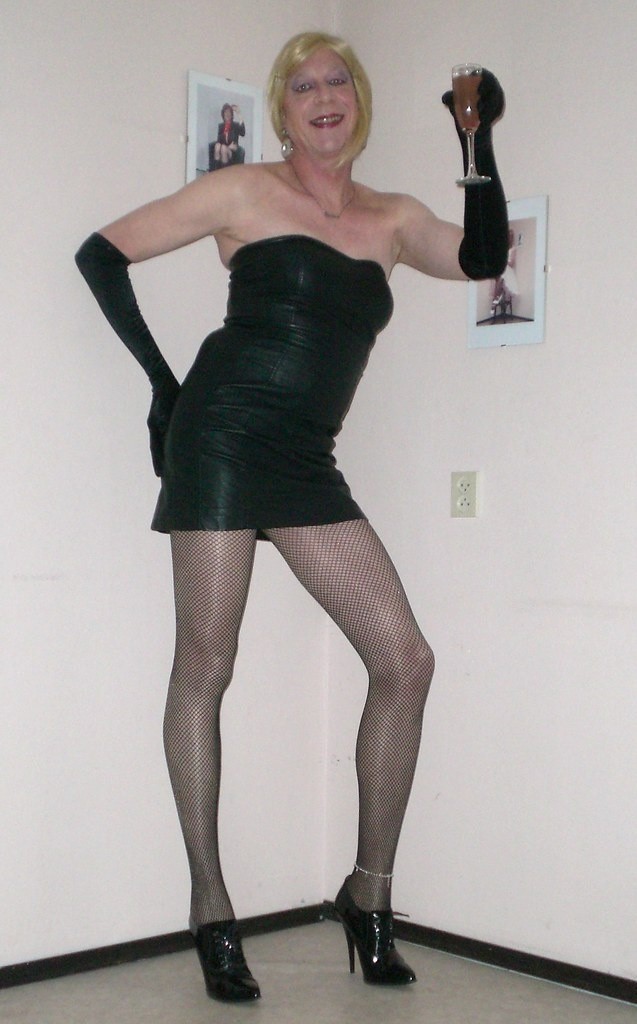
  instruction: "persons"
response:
[490,229,519,317]
[209,102,246,170]
[72,33,509,1004]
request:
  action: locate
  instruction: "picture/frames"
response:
[466,194,548,349]
[184,67,263,184]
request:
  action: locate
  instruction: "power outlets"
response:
[450,471,480,518]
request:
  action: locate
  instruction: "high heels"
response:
[193,918,262,1003]
[334,883,416,985]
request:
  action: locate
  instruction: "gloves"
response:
[443,71,510,279]
[74,232,181,475]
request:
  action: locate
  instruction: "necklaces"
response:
[287,157,356,218]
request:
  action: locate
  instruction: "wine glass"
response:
[452,63,492,185]
